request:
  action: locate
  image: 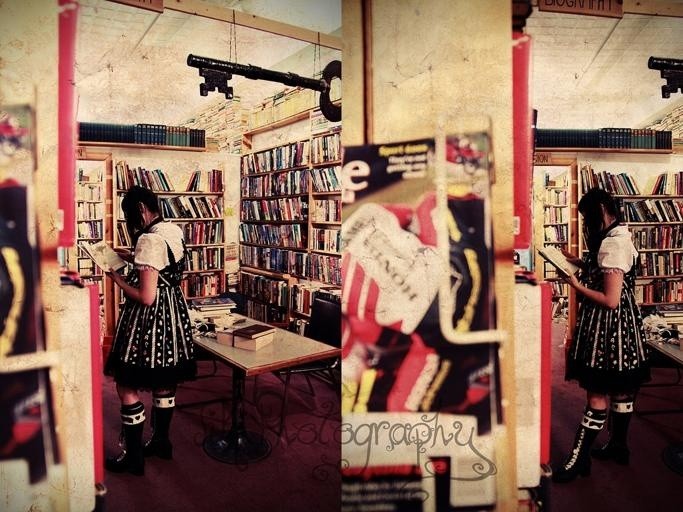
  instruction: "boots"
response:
[600,400,634,467]
[146,395,175,460]
[105,410,147,477]
[551,408,606,481]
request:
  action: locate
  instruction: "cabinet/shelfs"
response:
[59,127,343,512]
[514,170,683,499]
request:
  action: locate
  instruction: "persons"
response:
[550,186,653,484]
[101,186,197,475]
[338,190,486,411]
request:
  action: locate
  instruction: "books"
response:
[71,121,342,352]
[533,126,682,348]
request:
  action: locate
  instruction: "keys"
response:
[647,53,681,103]
[184,52,342,124]
